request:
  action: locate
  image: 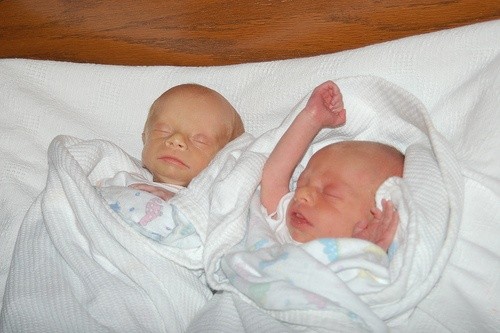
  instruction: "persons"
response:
[220,79,415,332]
[90,83,248,324]
